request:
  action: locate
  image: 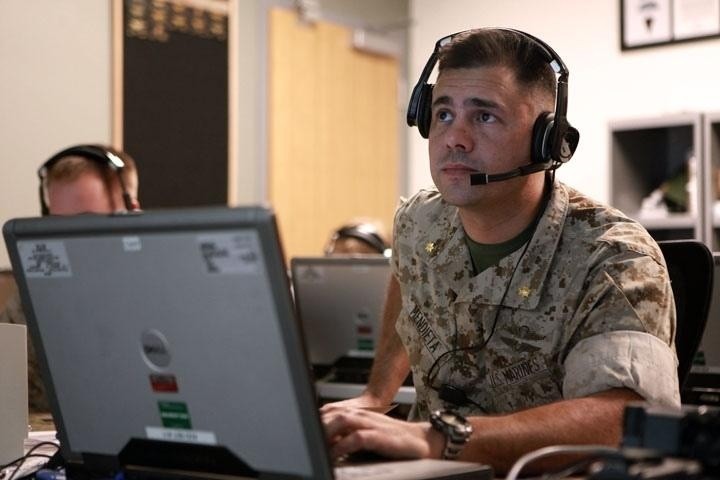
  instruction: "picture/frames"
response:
[617,0,716,55]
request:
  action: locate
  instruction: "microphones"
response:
[469,159,554,186]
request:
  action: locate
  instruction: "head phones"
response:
[407,27,579,168]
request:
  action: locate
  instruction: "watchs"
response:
[430,410,472,461]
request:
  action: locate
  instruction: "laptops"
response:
[3,205,494,480]
[290,255,394,369]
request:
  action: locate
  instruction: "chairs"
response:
[655,239,713,395]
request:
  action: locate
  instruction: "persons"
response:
[325,224,388,260]
[0,145,140,410]
[317,27,682,475]
